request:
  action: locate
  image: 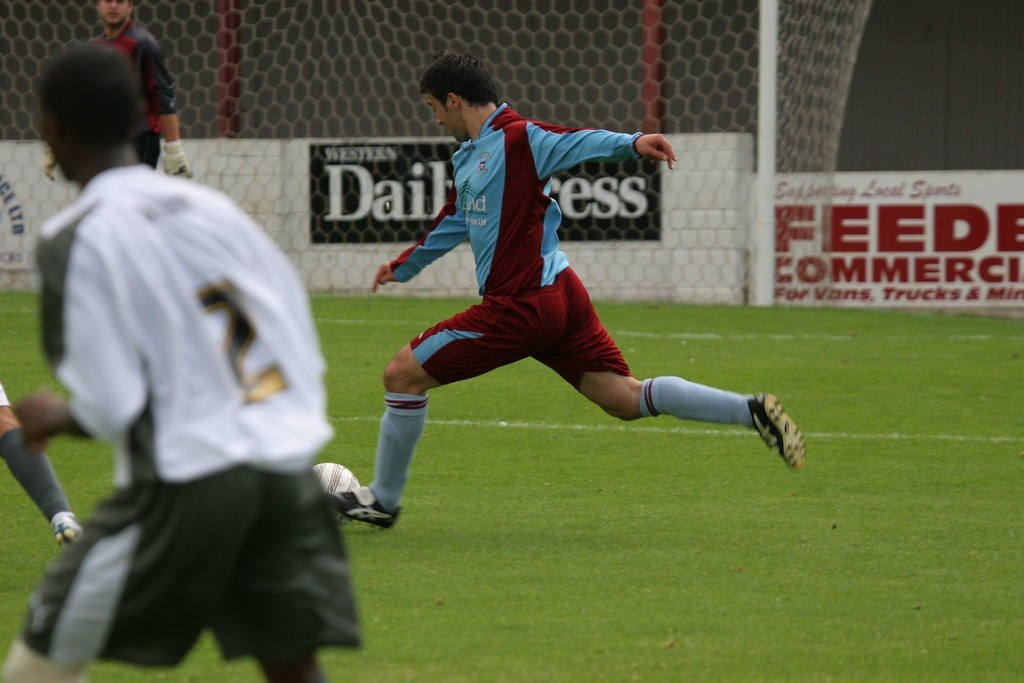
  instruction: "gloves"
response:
[43,147,57,181]
[164,144,194,180]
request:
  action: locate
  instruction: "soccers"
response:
[310,463,362,515]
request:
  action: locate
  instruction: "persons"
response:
[39,0,196,190]
[313,48,809,532]
[0,383,84,548]
[1,42,367,683]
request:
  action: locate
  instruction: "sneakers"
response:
[323,485,401,529]
[747,392,805,471]
[53,515,83,547]
